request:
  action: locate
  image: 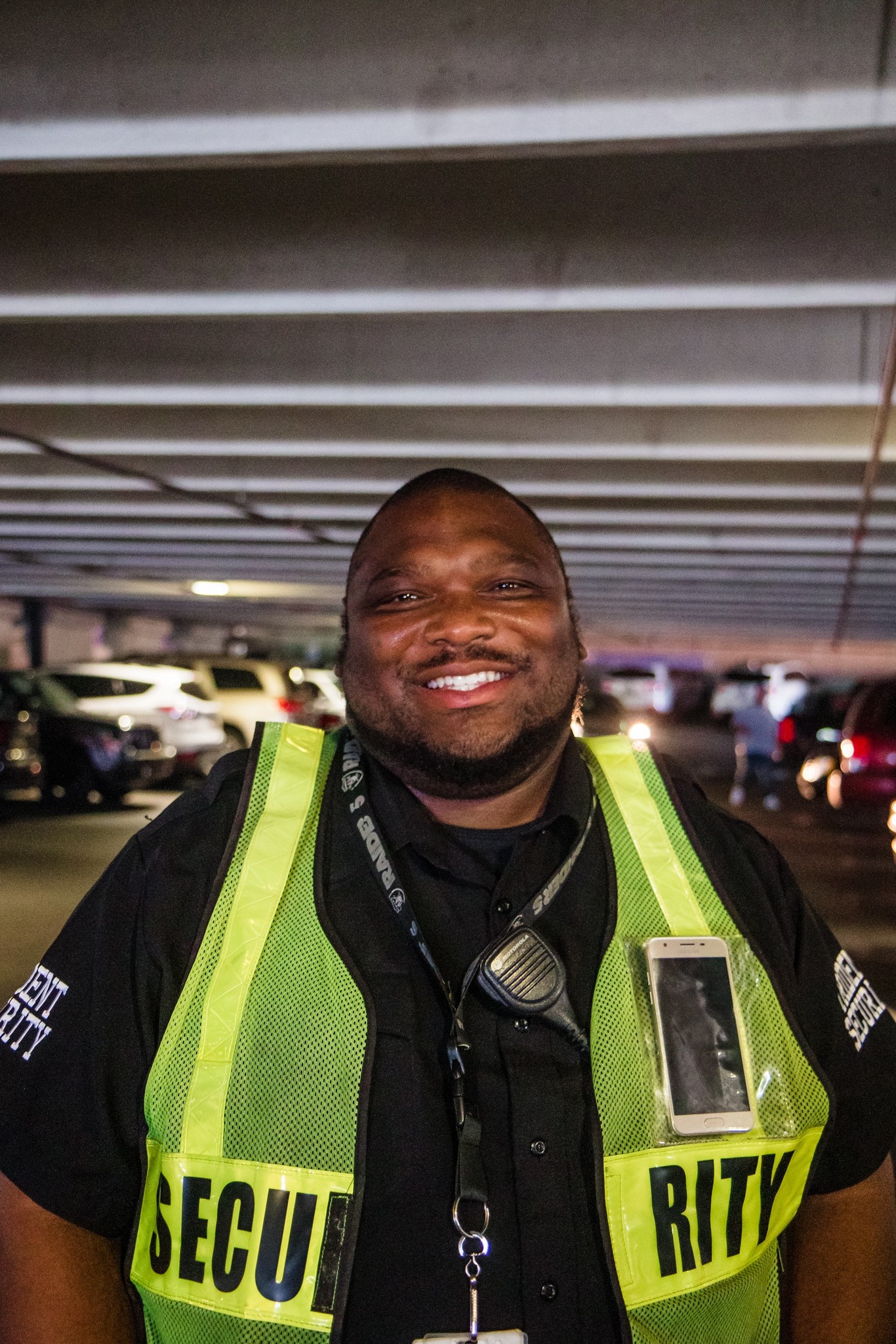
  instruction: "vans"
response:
[0,668,135,811]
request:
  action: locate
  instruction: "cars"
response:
[710,668,770,728]
[774,673,896,820]
[598,664,707,720]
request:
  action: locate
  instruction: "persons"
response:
[728,681,781,810]
[887,800,896,854]
[0,469,896,1344]
[795,686,851,808]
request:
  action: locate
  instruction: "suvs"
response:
[284,667,348,735]
[37,662,228,786]
[137,654,305,779]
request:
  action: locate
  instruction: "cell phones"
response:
[640,932,756,1133]
